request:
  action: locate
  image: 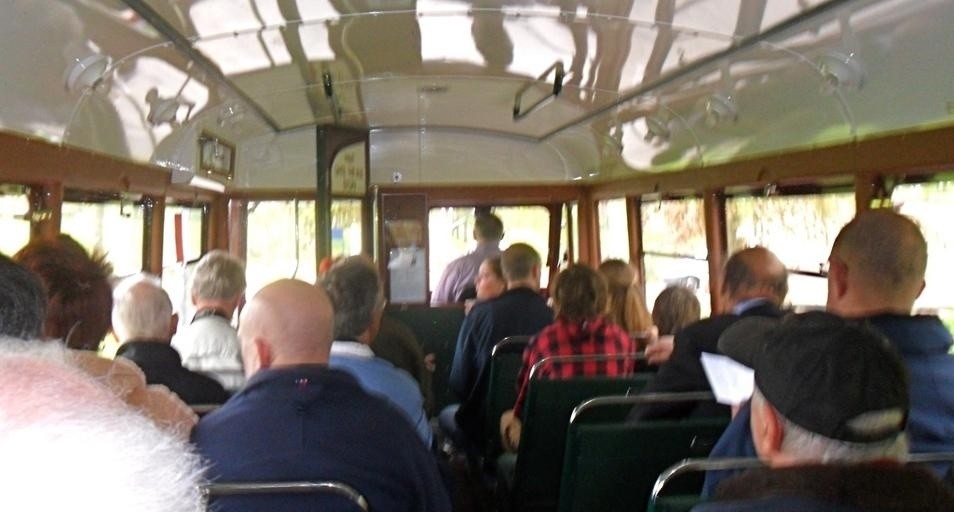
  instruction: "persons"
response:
[6,233,200,440]
[690,310,949,509]
[172,250,247,391]
[626,246,789,421]
[632,288,703,372]
[190,279,456,511]
[111,273,230,414]
[438,243,553,446]
[327,253,436,419]
[598,258,655,352]
[311,257,433,444]
[433,215,506,304]
[500,263,637,452]
[0,251,211,511]
[474,254,508,298]
[698,208,950,497]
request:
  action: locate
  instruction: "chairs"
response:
[649,450,954,512]
[180,402,220,414]
[500,351,651,497]
[562,392,731,511]
[473,334,534,460]
[204,481,372,512]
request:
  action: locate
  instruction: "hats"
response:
[717,306,911,444]
